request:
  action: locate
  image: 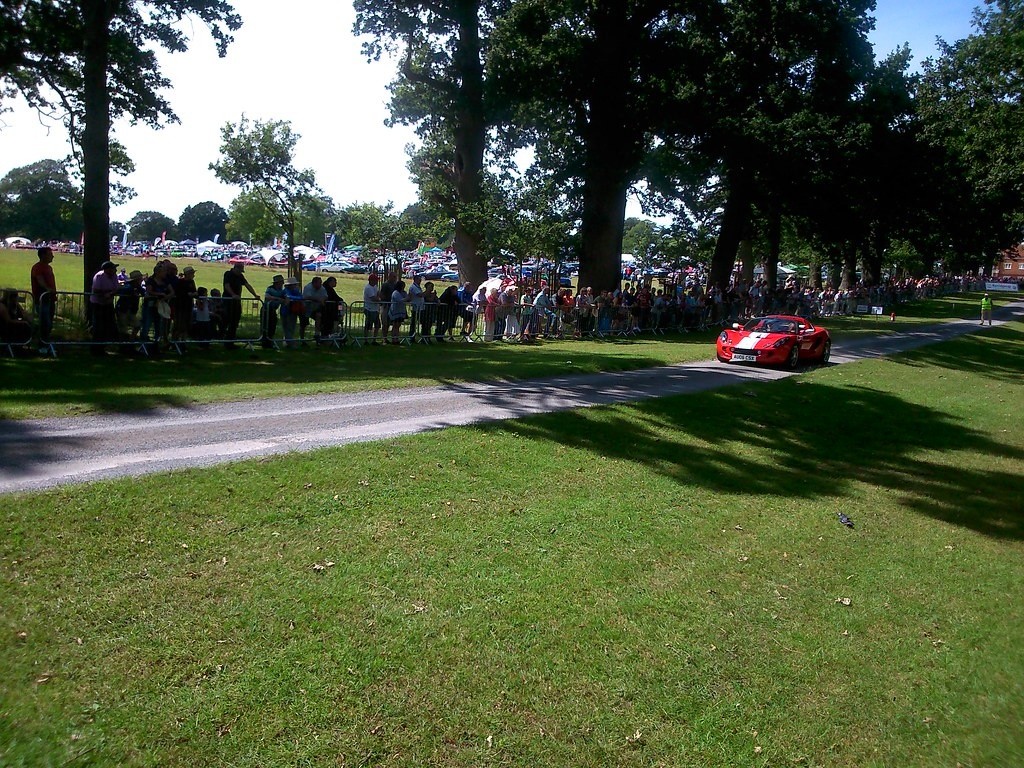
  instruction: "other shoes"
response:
[88,331,565,354]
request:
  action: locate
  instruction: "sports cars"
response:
[715,314,832,369]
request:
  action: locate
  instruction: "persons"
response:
[0,213,1024,379]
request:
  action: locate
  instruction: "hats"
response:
[128,270,145,281]
[368,274,379,280]
[182,267,197,275]
[284,277,299,285]
[101,261,119,270]
[425,282,434,287]
[234,262,245,273]
[272,275,284,283]
[154,263,169,273]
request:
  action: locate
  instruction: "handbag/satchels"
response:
[291,300,307,315]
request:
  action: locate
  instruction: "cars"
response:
[119,242,671,289]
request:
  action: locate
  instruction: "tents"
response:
[178,238,196,244]
[752,263,798,277]
[193,239,222,249]
[430,246,444,252]
[343,243,363,252]
[619,252,638,263]
[109,238,118,243]
